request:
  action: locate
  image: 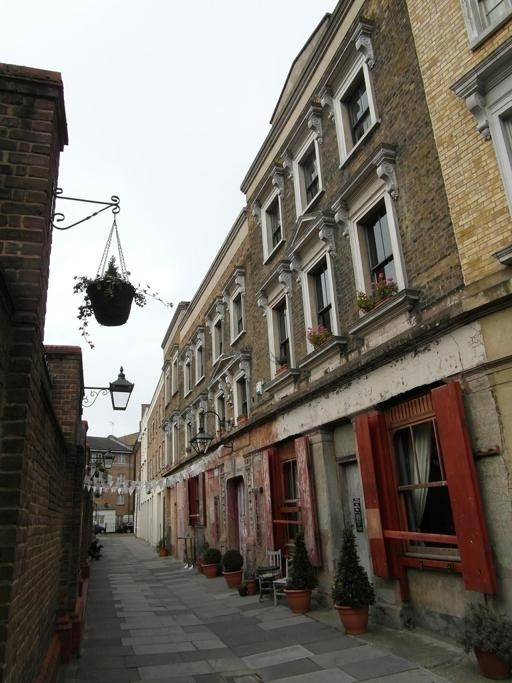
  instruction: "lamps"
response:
[81,363,135,411]
[188,410,234,456]
[89,447,116,470]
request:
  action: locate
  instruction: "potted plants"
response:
[270,352,289,375]
[200,548,222,577]
[460,599,512,680]
[282,528,317,616]
[220,549,245,588]
[331,527,378,636]
[155,537,169,557]
[73,213,174,350]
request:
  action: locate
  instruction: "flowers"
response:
[354,271,399,309]
[303,322,334,344]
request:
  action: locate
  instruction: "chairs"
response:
[256,547,283,604]
[272,557,293,605]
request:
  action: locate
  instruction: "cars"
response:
[93,519,105,534]
[119,522,134,533]
